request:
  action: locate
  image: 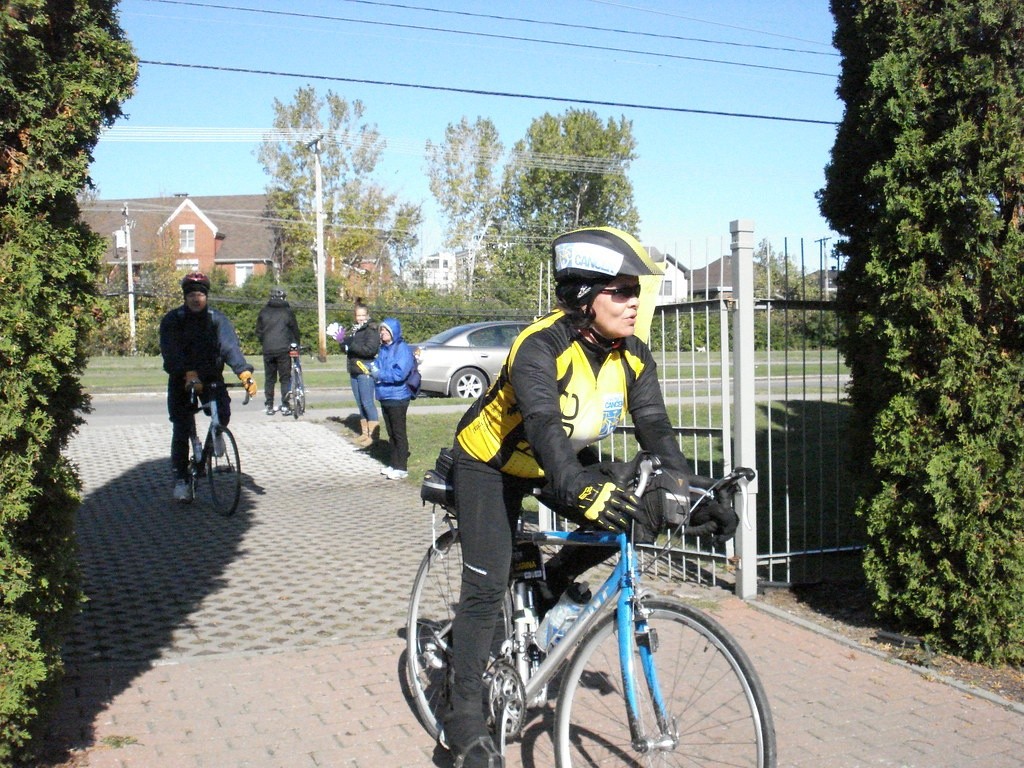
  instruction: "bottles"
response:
[193,438,202,463]
[536,582,592,653]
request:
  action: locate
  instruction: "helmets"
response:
[270,289,286,302]
[181,272,210,291]
[549,226,665,279]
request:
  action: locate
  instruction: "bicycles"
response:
[406,449,778,768]
[186,376,253,516]
[285,343,312,419]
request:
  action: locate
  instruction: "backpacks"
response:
[394,341,422,400]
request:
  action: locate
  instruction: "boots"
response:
[356,419,380,447]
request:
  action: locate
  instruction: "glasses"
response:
[600,284,641,298]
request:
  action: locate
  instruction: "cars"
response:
[406,321,534,398]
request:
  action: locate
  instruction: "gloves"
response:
[240,370,258,397]
[572,476,646,535]
[691,504,740,548]
[186,372,203,392]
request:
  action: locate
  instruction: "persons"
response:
[340,297,380,448]
[432,226,739,768]
[160,272,258,503]
[364,318,415,480]
[255,288,300,416]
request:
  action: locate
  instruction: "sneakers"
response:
[174,477,192,503]
[281,407,293,415]
[381,466,409,480]
[211,433,225,458]
[438,722,504,767]
[266,406,274,415]
